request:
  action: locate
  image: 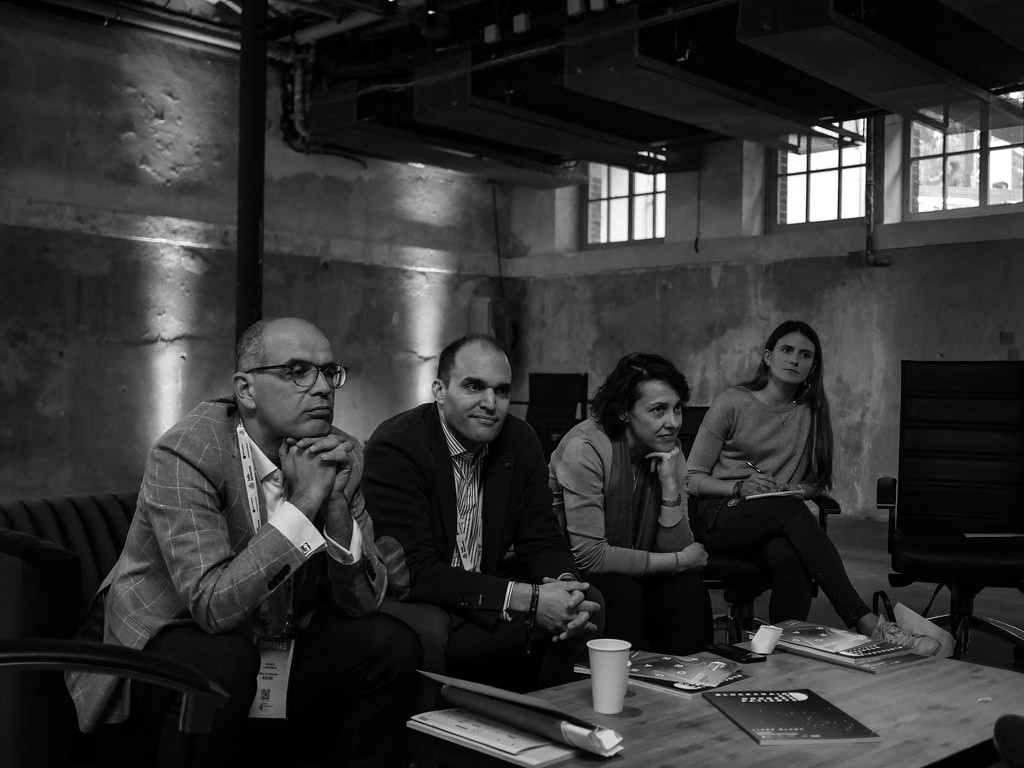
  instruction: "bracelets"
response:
[727,481,744,506]
[526,583,540,630]
[796,483,804,499]
[675,552,679,573]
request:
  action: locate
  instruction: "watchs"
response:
[661,493,681,507]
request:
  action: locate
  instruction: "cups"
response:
[623,660,632,697]
[587,639,631,714]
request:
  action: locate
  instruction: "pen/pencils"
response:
[746,461,782,488]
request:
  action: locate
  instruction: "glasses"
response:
[245,361,350,389]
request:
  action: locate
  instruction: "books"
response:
[572,651,881,746]
[745,619,934,675]
[405,670,615,768]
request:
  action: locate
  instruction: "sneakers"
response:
[870,612,941,656]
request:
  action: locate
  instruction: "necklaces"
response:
[762,389,796,427]
[632,471,636,482]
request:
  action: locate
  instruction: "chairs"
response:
[526,373,589,465]
[0,492,454,768]
[676,407,842,644]
[877,360,1024,661]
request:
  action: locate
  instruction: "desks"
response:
[411,640,1024,768]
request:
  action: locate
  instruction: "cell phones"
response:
[706,643,767,664]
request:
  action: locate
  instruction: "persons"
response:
[360,333,607,680]
[545,351,714,659]
[65,315,424,768]
[686,319,940,660]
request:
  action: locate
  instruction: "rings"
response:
[757,485,761,492]
